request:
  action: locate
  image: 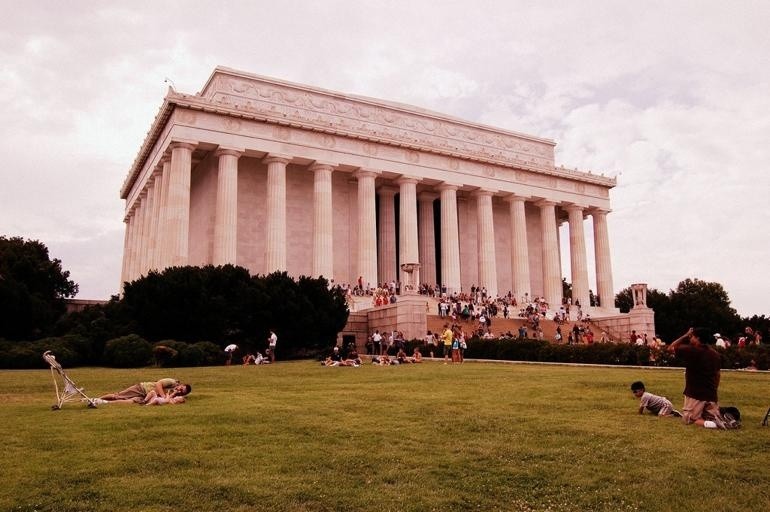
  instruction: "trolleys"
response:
[43,351,99,410]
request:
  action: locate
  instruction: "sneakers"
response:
[715,412,741,430]
[670,410,683,418]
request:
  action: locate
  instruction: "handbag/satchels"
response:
[432,338,438,347]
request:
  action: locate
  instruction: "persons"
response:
[91,378,191,404]
[630,330,661,348]
[667,323,740,430]
[713,326,762,348]
[139,389,186,405]
[320,275,611,367]
[631,380,683,418]
[224,330,277,365]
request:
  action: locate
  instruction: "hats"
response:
[713,333,721,337]
